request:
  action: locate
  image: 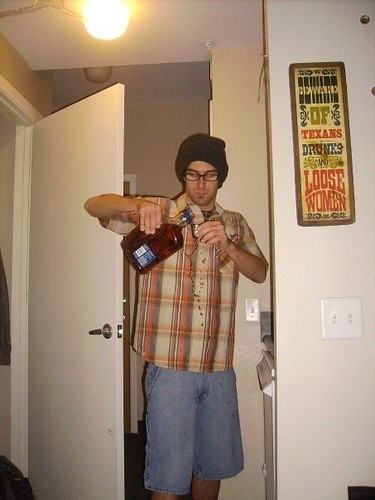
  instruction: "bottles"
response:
[120,208,195,275]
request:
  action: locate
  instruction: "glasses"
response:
[182,168,219,181]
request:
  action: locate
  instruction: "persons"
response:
[84,133,268,500]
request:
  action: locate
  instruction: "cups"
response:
[192,224,202,238]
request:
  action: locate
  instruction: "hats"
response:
[174,132,229,190]
